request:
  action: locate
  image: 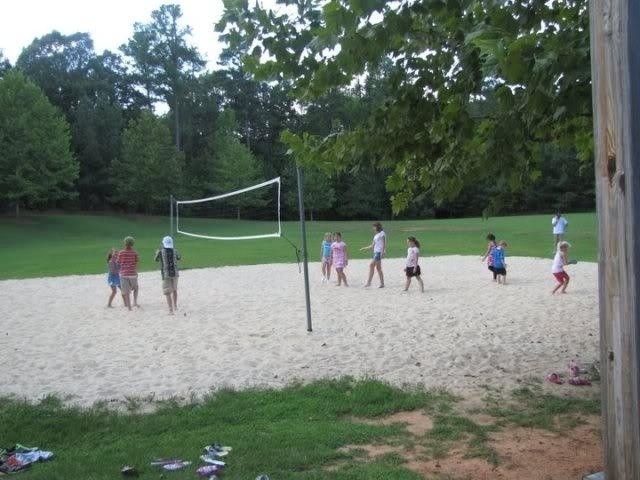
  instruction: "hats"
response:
[162,236,174,249]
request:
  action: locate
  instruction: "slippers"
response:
[256,475,269,480]
[121,464,137,475]
[151,458,192,470]
[196,443,232,480]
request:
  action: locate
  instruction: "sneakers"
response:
[0,446,53,472]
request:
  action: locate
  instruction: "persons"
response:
[402,236,424,294]
[552,209,568,255]
[491,240,507,285]
[107,249,128,308]
[551,241,577,294]
[330,231,350,287]
[114,235,140,311]
[482,233,498,281]
[320,232,333,283]
[360,222,387,288]
[154,236,181,315]
